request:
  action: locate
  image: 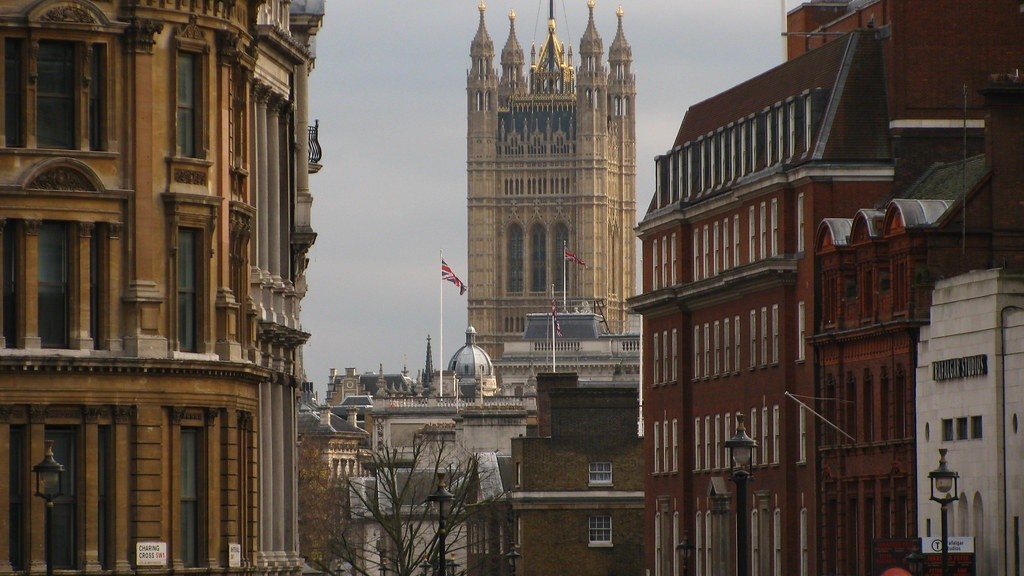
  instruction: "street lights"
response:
[928,450,960,576]
[425,481,456,576]
[723,414,761,576]
[33,440,65,576]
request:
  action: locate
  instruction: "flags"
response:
[441,258,467,296]
[551,299,563,338]
[564,246,588,268]
